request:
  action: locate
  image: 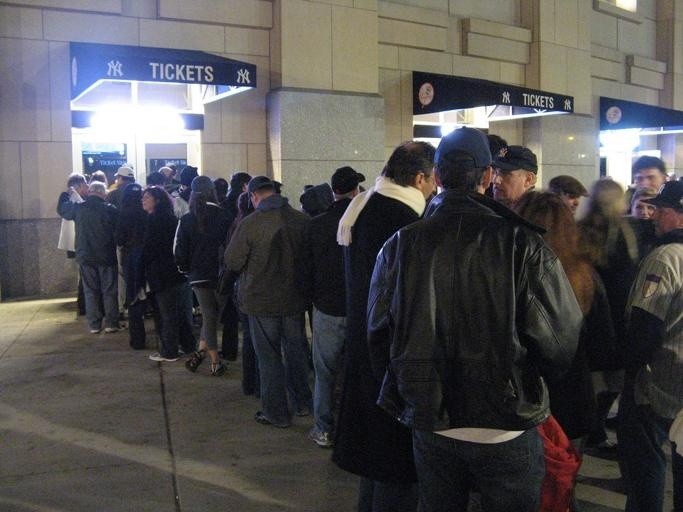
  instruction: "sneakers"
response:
[90,329,101,335]
[255,411,290,428]
[296,404,311,417]
[148,351,179,362]
[308,428,336,447]
[105,323,127,333]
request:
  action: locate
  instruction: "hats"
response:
[190,175,215,193]
[185,349,207,372]
[433,126,492,168]
[246,176,270,210]
[332,166,367,195]
[638,180,683,209]
[549,174,591,197]
[113,166,135,178]
[211,360,227,377]
[490,145,538,176]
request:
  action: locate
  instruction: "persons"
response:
[177,167,200,204]
[629,184,660,222]
[630,156,670,195]
[127,185,198,363]
[228,192,265,398]
[364,125,583,512]
[490,145,539,220]
[104,165,135,322]
[112,183,163,351]
[172,176,229,378]
[613,180,683,511]
[57,181,120,335]
[513,192,612,512]
[572,179,654,451]
[547,174,589,218]
[332,139,437,511]
[218,172,251,362]
[223,175,313,429]
[57,174,91,317]
[299,166,366,451]
